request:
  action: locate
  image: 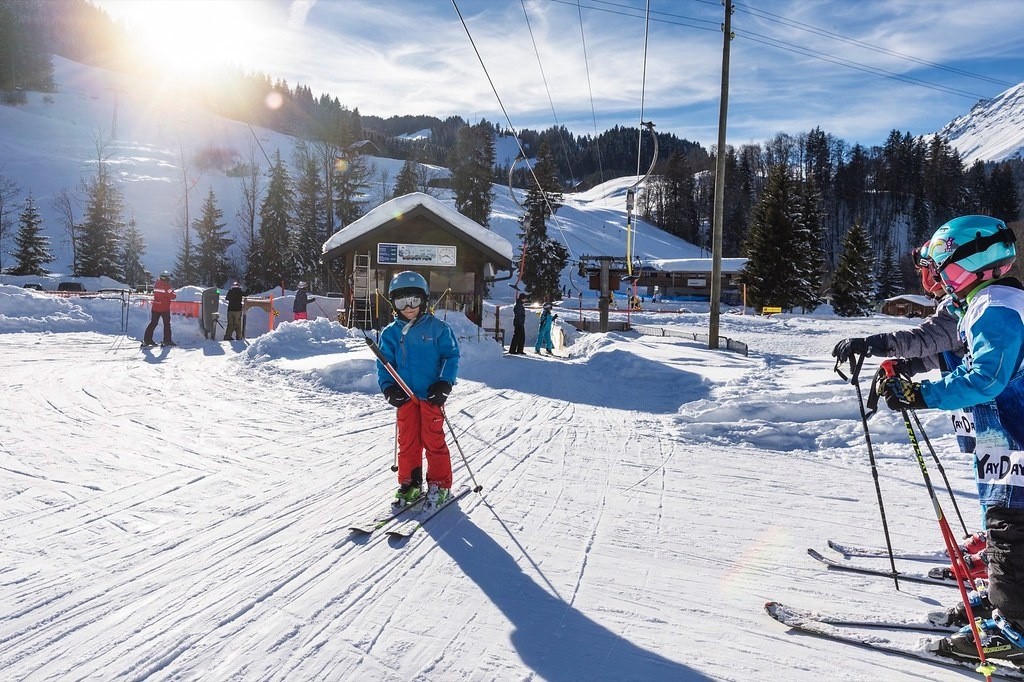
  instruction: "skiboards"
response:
[346,484,472,538]
[764,539,1024,682]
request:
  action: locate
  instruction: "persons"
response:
[879,215,1024,663]
[143,270,177,346]
[535,300,557,356]
[293,282,315,320]
[562,285,656,310]
[509,293,527,355]
[224,282,251,341]
[375,271,460,510]
[832,240,990,583]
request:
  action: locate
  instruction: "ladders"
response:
[347,250,373,330]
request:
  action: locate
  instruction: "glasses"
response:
[391,292,424,311]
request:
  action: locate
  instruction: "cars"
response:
[23,283,47,291]
[58,282,87,292]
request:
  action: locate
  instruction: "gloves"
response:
[426,382,453,407]
[879,377,928,411]
[832,333,889,363]
[383,386,406,407]
[877,357,928,379]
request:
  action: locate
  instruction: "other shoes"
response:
[546,351,553,355]
[509,351,518,355]
[223,335,233,340]
[144,340,157,346]
[163,340,175,345]
[535,350,541,355]
[517,351,526,355]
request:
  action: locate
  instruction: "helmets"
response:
[928,215,1017,300]
[161,270,170,283]
[297,281,309,292]
[543,301,553,309]
[231,282,241,288]
[389,271,430,298]
[912,240,943,292]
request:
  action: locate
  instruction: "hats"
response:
[519,293,526,300]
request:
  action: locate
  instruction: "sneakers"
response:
[965,530,988,553]
[425,484,449,504]
[950,615,1024,668]
[395,484,423,499]
[950,547,988,580]
[948,590,998,624]
